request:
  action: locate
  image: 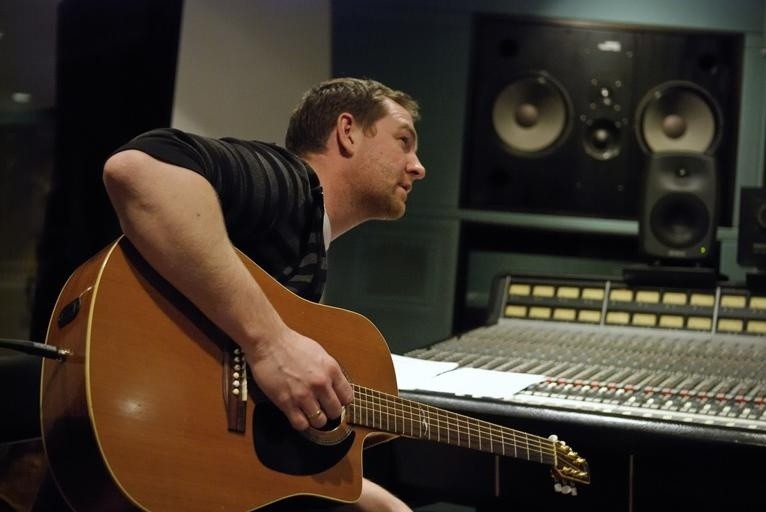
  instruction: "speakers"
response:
[736,186,766,266]
[457,14,746,227]
[640,150,716,262]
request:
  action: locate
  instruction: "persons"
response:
[30,76,427,512]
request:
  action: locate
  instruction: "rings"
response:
[306,409,322,418]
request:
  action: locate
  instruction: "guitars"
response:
[38,232,592,511]
